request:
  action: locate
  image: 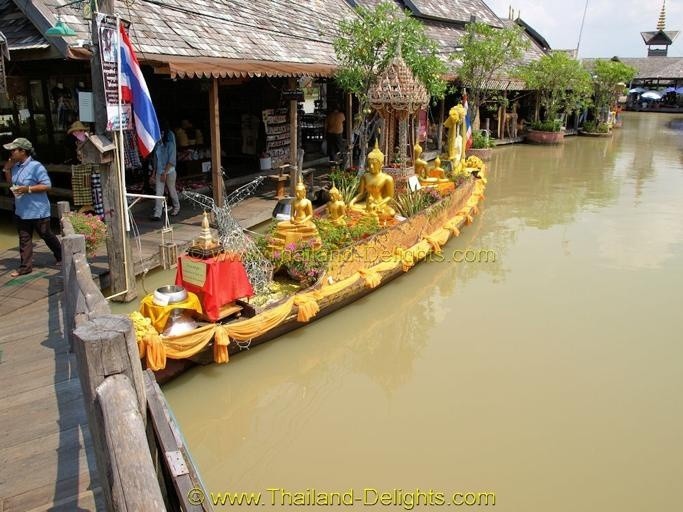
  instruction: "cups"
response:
[10,185,23,199]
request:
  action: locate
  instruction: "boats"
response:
[128,157,488,387]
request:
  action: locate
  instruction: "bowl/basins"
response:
[154,285,188,303]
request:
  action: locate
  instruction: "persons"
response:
[323,181,346,228]
[276,175,316,232]
[323,102,344,155]
[405,143,430,185]
[347,137,395,218]
[1,136,60,277]
[430,154,445,179]
[148,117,180,219]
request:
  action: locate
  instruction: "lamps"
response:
[44,0,92,37]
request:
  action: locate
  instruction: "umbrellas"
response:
[628,85,682,100]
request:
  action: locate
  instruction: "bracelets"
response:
[2,168,10,172]
[27,185,32,195]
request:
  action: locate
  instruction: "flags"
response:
[120,23,162,158]
[461,100,473,152]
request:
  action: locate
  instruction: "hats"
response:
[66,121,89,135]
[2,138,32,152]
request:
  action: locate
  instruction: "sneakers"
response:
[55,261,62,266]
[11,269,31,277]
[151,206,179,219]
